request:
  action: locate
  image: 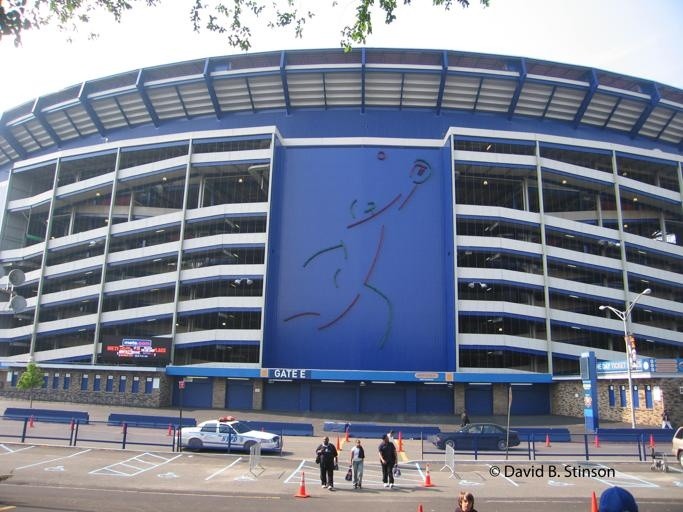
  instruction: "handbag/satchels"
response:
[315,454,321,464]
[393,468,401,478]
[345,469,353,481]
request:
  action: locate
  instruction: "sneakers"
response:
[384,483,394,488]
[353,483,361,489]
[321,485,333,490]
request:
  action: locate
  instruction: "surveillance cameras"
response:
[360,381,366,387]
[448,384,454,388]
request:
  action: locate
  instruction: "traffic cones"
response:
[397,431,404,452]
[589,491,599,512]
[29,414,34,427]
[545,432,552,447]
[295,472,310,498]
[334,435,342,451]
[649,434,657,449]
[594,434,600,448]
[166,423,173,436]
[343,431,351,443]
[423,463,433,487]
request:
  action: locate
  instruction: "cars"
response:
[671,425,683,469]
[432,423,520,450]
[175,416,283,453]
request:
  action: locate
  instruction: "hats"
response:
[599,485,639,512]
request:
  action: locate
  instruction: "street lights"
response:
[599,287,652,429]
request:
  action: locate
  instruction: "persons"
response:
[377,434,398,488]
[315,436,338,490]
[660,409,673,430]
[461,411,470,427]
[345,440,364,489]
[454,492,478,512]
